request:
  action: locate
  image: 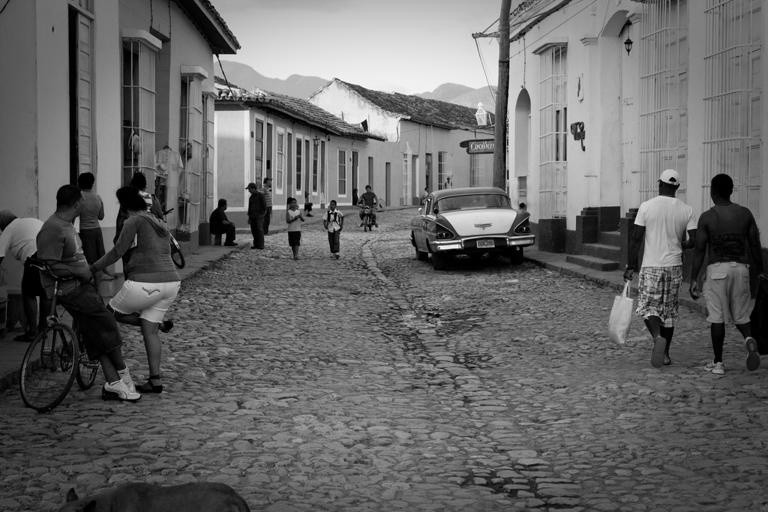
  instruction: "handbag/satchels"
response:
[751,277,767,354]
[608,282,634,345]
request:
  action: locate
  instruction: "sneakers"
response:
[650,336,670,367]
[14,333,40,342]
[161,320,173,334]
[134,382,163,394]
[102,379,142,401]
[359,221,378,228]
[117,367,137,392]
[224,241,238,246]
[703,360,726,375]
[745,337,760,371]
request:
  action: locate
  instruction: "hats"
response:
[245,183,256,189]
[657,169,680,186]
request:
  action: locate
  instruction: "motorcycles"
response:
[355,203,374,233]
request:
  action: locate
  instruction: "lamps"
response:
[624,15,633,55]
[475,101,497,128]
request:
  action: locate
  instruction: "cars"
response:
[408,183,536,268]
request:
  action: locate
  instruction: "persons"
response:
[688,174,768,377]
[422,186,431,205]
[620,168,699,366]
[286,198,304,261]
[257,178,273,236]
[244,183,268,251]
[209,199,239,247]
[322,200,344,259]
[1,171,185,403]
[304,191,313,217]
[444,177,453,190]
[357,185,381,228]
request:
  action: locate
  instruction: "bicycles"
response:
[158,207,188,270]
[16,260,100,414]
